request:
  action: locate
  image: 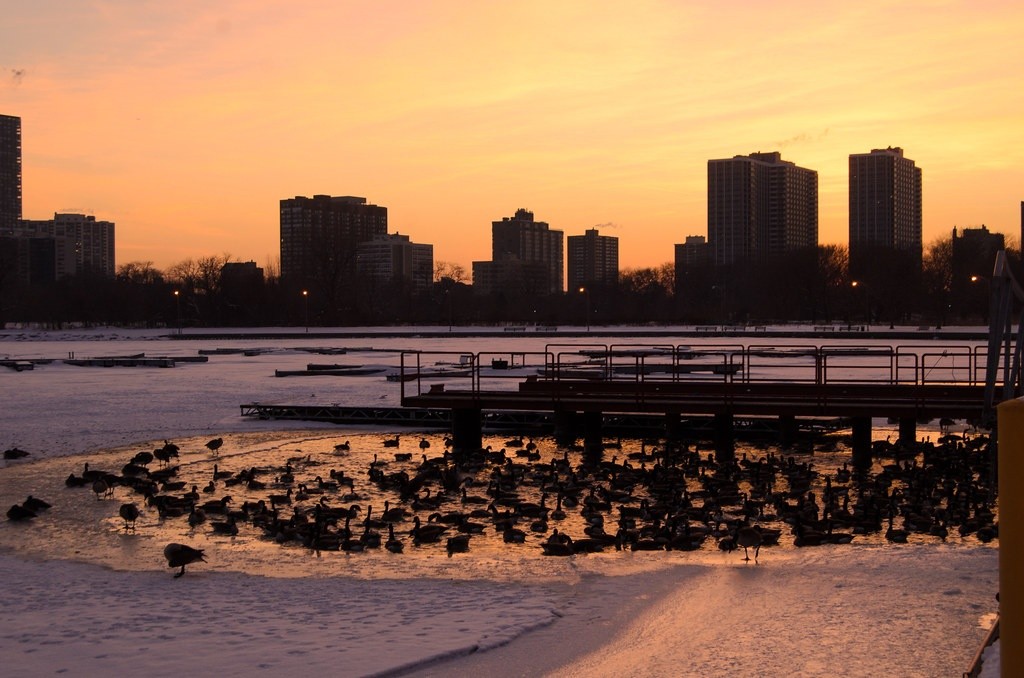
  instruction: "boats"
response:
[318,347,346,355]
[243,349,273,356]
[385,373,416,383]
[274,362,477,378]
[537,365,614,380]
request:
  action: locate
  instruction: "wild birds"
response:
[6,495,53,521]
[3,447,30,459]
[164,543,209,578]
[65,428,999,560]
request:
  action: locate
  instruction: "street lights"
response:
[175,291,182,334]
[579,288,590,331]
[303,291,308,332]
[852,280,870,331]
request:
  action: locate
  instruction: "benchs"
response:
[695,326,766,331]
[536,327,557,332]
[814,326,834,332]
[839,326,859,331]
[504,327,526,332]
